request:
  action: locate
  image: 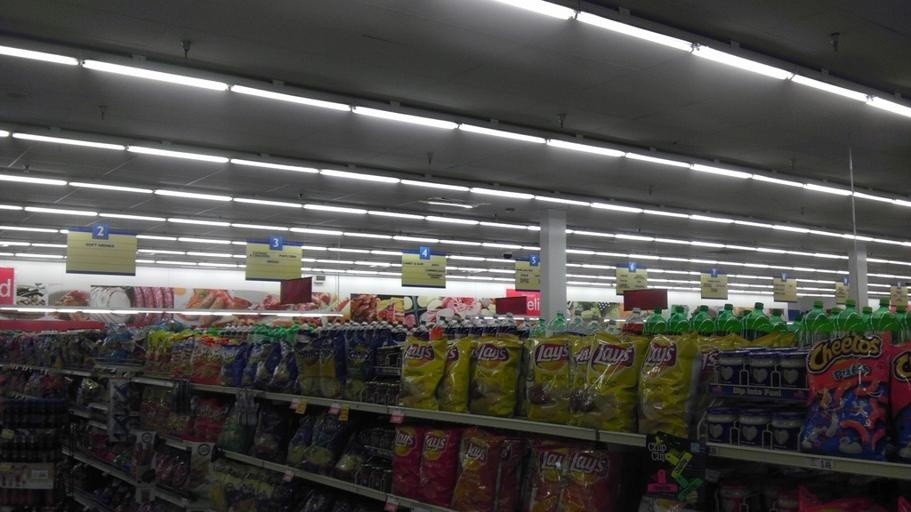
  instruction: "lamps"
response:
[0,0,911,322]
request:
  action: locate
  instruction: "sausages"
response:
[132,286,174,328]
[184,293,214,320]
[199,298,224,326]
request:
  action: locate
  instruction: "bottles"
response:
[0,402,72,511]
[189,296,911,346]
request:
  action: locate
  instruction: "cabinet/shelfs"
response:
[0,353,911,511]
